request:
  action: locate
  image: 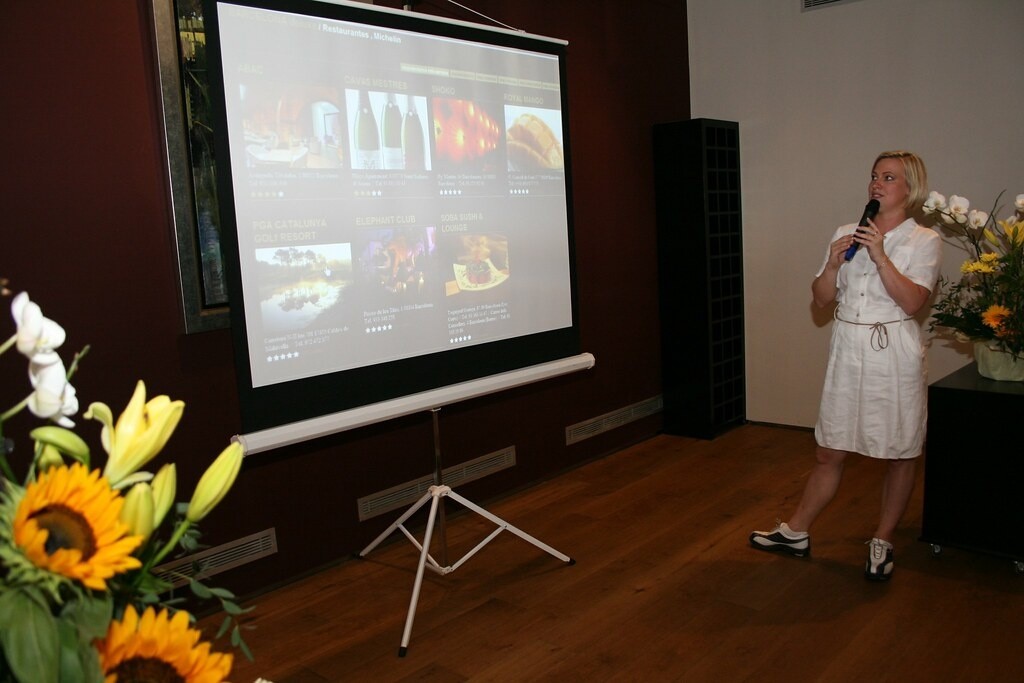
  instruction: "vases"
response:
[972,338,1024,382]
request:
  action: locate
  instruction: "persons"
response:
[749,148,942,583]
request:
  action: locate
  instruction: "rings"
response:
[872,231,878,236]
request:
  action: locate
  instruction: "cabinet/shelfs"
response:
[916,361,1024,576]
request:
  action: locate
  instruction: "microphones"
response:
[845,199,880,262]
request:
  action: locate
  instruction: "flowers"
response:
[917,187,1024,363]
[0,292,258,683]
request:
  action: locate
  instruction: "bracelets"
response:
[876,257,891,271]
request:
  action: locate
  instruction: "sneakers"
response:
[748,530,809,558]
[864,538,894,583]
